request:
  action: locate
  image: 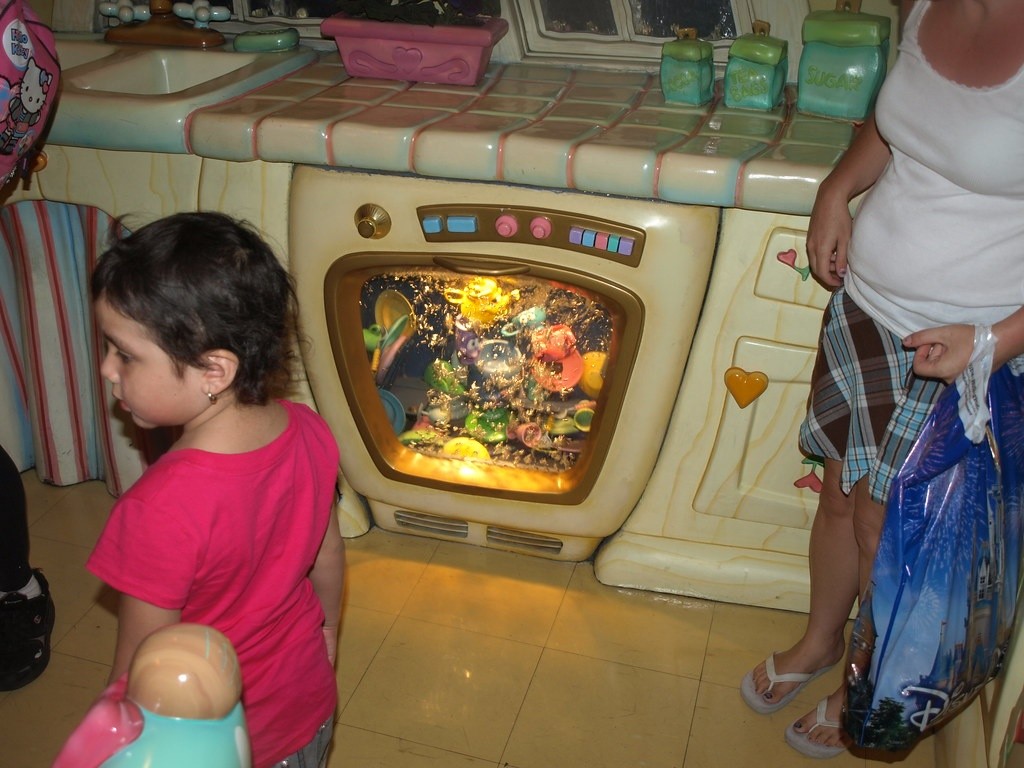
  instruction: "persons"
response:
[735,1,1024,757]
[83,210,348,768]
[0,444,56,692]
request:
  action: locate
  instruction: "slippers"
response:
[741,650,824,715]
[785,696,848,758]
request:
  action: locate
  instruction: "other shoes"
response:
[0,570,55,691]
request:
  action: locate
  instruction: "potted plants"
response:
[321,0,509,86]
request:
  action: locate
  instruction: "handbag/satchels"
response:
[0,0,60,189]
[838,323,1024,763]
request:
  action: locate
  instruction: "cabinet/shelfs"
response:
[594,209,859,626]
